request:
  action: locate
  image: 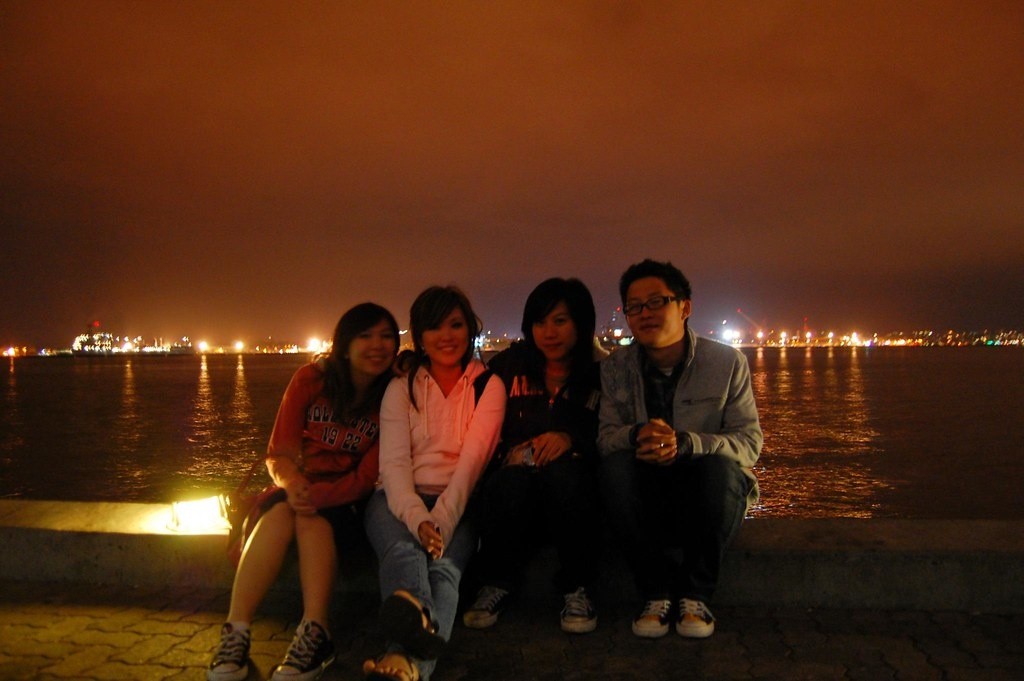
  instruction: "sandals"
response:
[365,642,425,681]
[378,593,446,662]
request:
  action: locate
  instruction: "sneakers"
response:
[560,587,597,632]
[631,600,672,638]
[207,623,250,681]
[463,583,508,628]
[675,599,717,638]
[270,620,336,681]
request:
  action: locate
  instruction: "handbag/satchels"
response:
[224,452,286,573]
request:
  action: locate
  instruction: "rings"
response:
[658,441,663,447]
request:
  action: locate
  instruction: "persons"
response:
[464,278,598,631]
[206,304,401,681]
[596,260,765,638]
[362,287,506,681]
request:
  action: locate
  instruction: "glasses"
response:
[623,296,682,316]
